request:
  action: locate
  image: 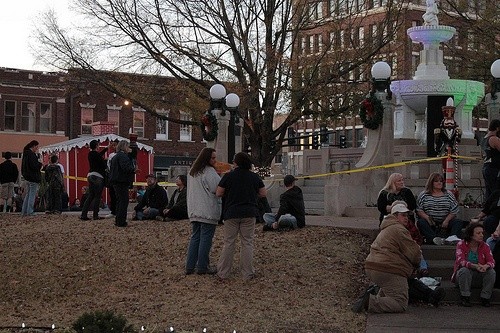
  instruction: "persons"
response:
[186,148,220,276]
[263,175,305,231]
[434,97,462,188]
[132,174,168,220]
[216,152,266,282]
[391,201,430,276]
[0,152,69,214]
[378,173,415,229]
[452,223,496,307]
[80,140,112,220]
[476,119,500,289]
[71,199,82,211]
[21,140,43,215]
[81,185,88,207]
[155,175,189,222]
[416,172,462,246]
[106,140,133,227]
[352,204,421,314]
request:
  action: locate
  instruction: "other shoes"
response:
[482,297,490,306]
[353,290,369,313]
[263,225,272,231]
[185,267,196,273]
[462,296,473,308]
[196,266,216,274]
[29,212,36,216]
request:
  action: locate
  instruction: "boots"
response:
[82,209,91,220]
[93,210,105,220]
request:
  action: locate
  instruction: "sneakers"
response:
[433,237,445,245]
[445,235,462,245]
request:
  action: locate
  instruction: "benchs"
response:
[414,245,500,302]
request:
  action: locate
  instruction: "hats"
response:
[284,175,298,182]
[145,174,156,179]
[391,204,409,214]
[90,139,100,148]
